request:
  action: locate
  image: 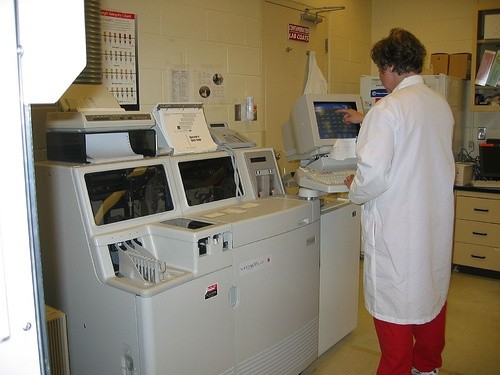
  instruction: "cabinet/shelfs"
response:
[470,0,500,112]
[452,189,500,273]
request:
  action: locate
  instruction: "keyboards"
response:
[308,169,357,184]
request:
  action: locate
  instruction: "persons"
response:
[332,27,456,375]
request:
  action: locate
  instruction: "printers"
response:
[45,82,157,166]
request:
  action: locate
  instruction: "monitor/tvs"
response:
[281,93,364,163]
